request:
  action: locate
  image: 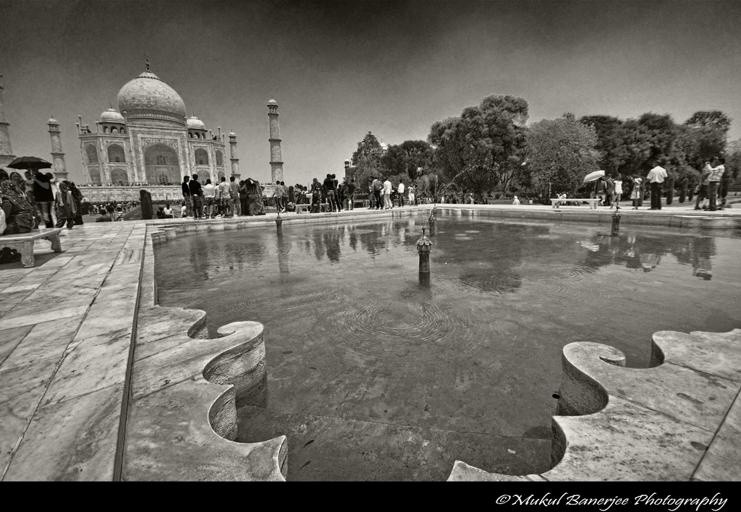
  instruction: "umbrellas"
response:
[582,169,605,183]
[5,154,52,176]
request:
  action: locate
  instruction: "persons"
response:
[0,167,84,229]
[592,172,645,210]
[553,191,563,208]
[512,195,522,205]
[645,157,731,211]
[560,193,567,205]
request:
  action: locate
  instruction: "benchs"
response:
[550,199,602,209]
[1,227,61,268]
